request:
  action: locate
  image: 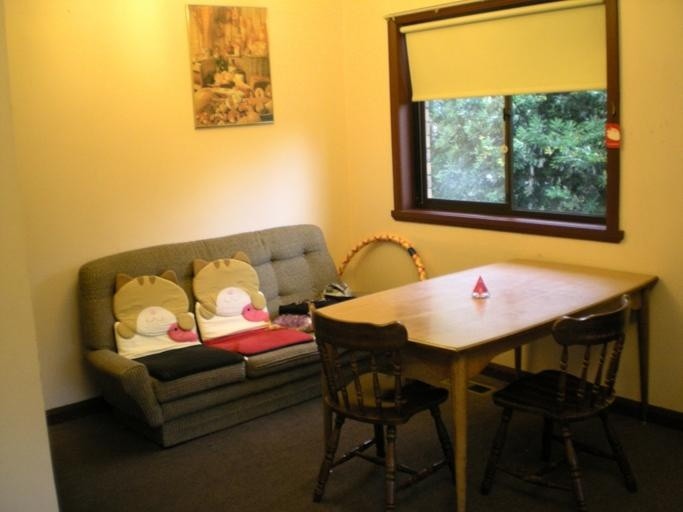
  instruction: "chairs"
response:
[310,311,456,512]
[479,293,639,512]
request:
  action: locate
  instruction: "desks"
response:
[314,260,659,511]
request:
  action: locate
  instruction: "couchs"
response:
[78,223,353,449]
